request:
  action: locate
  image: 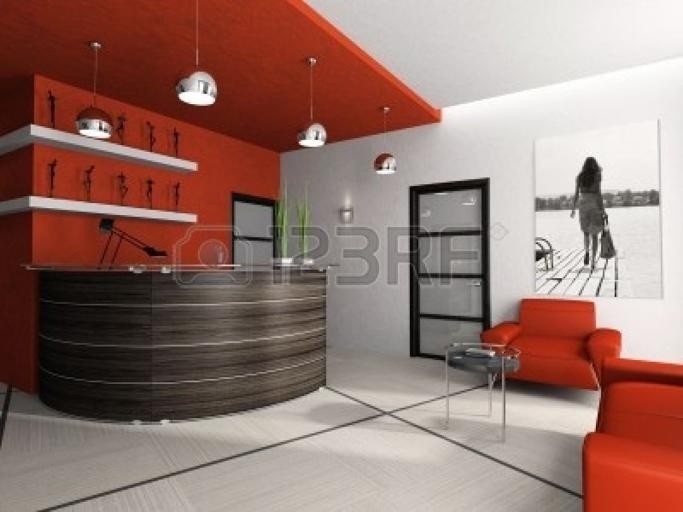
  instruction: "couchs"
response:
[581,356,683,512]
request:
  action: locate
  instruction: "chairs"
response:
[479,298,622,397]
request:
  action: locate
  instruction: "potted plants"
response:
[270,177,315,266]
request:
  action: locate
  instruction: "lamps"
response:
[175,0,217,107]
[373,106,397,176]
[296,57,327,148]
[74,40,114,139]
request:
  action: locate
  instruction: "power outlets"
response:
[96,217,167,265]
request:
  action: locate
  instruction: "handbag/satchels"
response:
[600,214,617,259]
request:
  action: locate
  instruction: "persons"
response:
[569,154,611,273]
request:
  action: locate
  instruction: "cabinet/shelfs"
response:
[0,124,198,225]
